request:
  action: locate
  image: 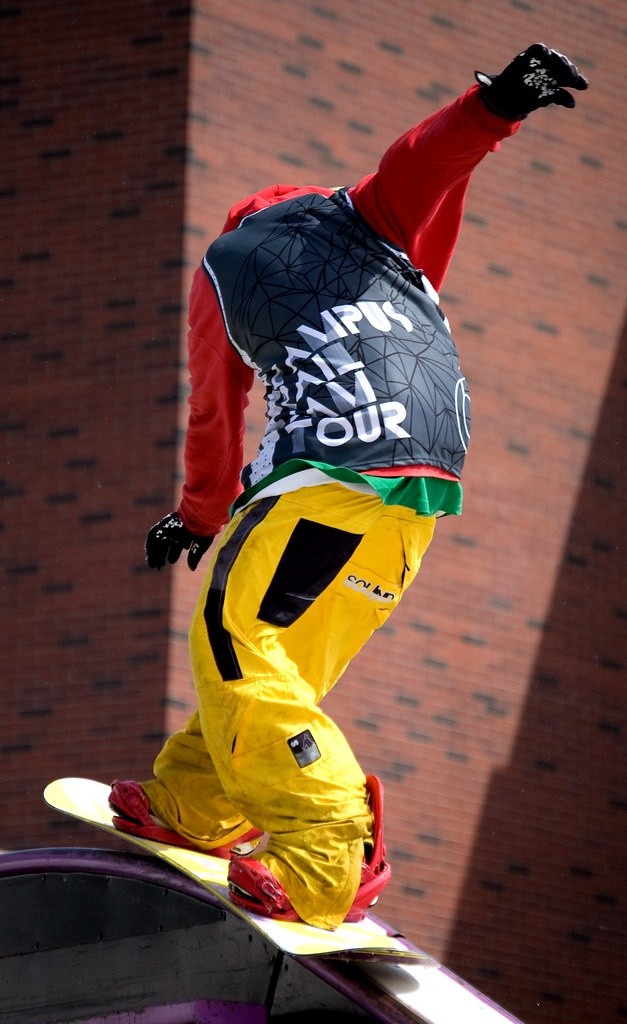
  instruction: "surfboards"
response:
[41,772,434,966]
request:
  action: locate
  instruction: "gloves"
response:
[474,43,587,122]
[145,512,216,571]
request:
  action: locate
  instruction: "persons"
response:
[107,43,590,930]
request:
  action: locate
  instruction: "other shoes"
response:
[229,774,386,923]
[109,780,272,859]
